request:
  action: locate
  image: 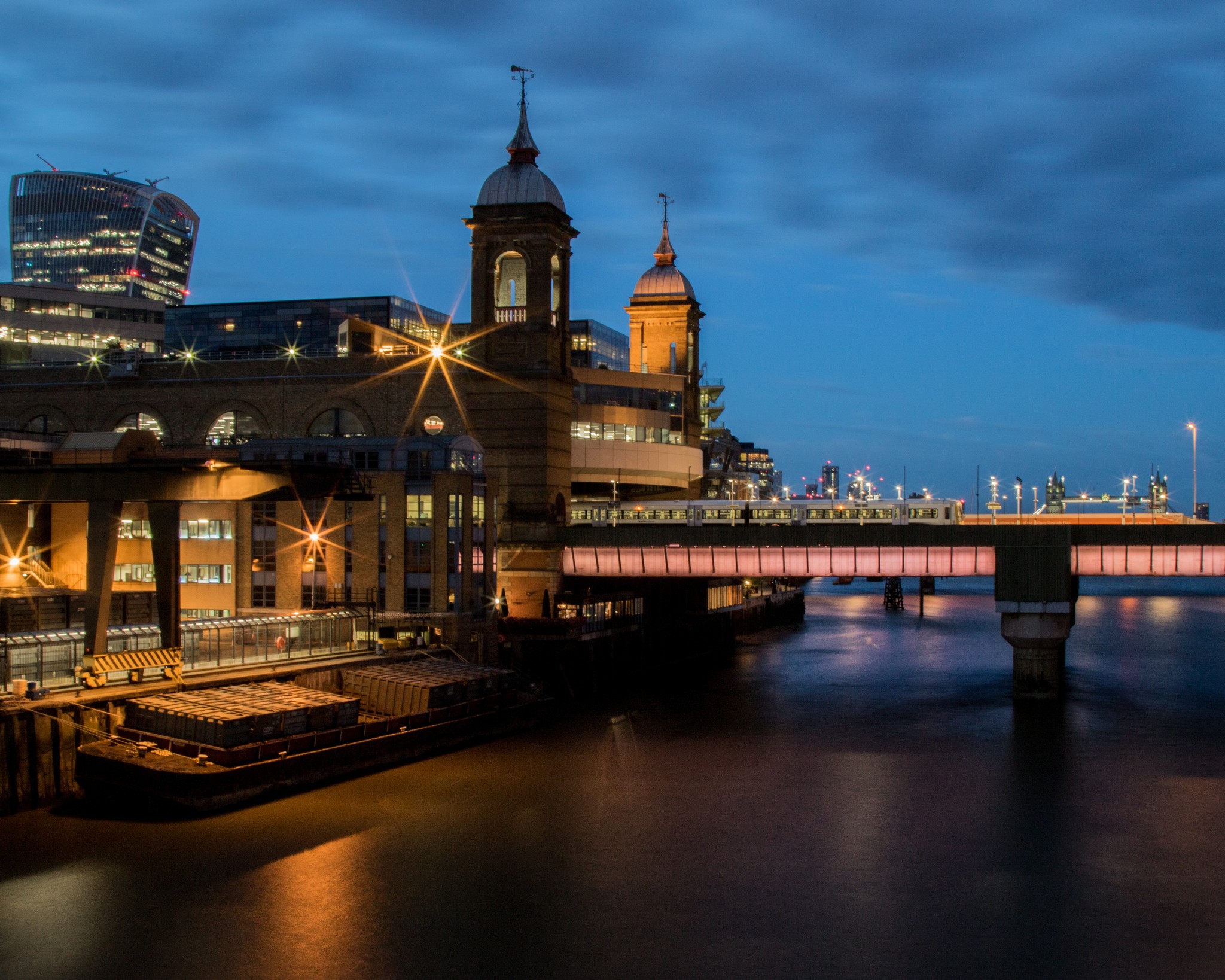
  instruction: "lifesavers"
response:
[277,637,285,649]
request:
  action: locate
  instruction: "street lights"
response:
[858,477,863,526]
[308,532,320,612]
[688,466,692,508]
[829,490,833,523]
[1016,486,1022,519]
[729,479,736,527]
[747,483,758,499]
[1033,487,1038,512]
[923,488,928,498]
[990,477,999,525]
[1123,480,1129,524]
[611,480,619,527]
[896,486,902,499]
[1003,495,1006,513]
[1188,424,1198,520]
[866,483,871,498]
[1132,475,1137,525]
[783,487,789,500]
